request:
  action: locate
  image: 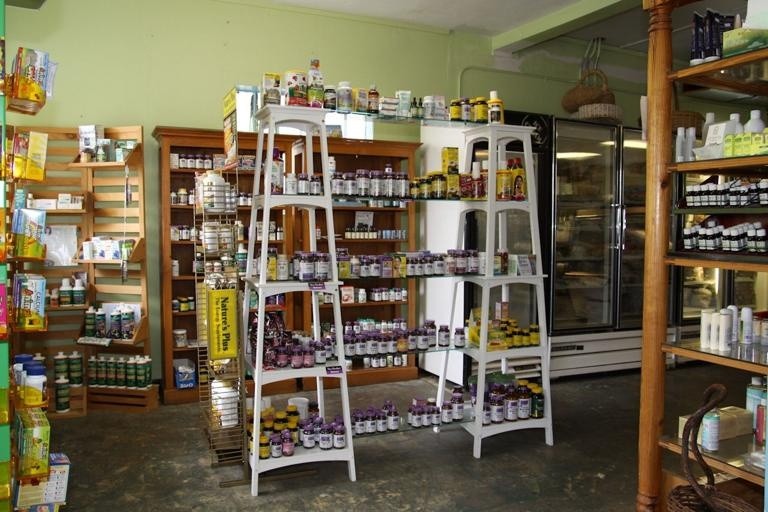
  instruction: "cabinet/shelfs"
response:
[636,0,766,512]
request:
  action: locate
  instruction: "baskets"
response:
[578,104,623,124]
[666,383,762,512]
[672,81,705,139]
[562,70,615,113]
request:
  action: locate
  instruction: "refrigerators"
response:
[552,115,731,373]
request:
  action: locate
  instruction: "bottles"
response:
[344,225,408,240]
[681,218,768,254]
[249,312,541,368]
[746,374,768,447]
[353,289,408,302]
[698,302,767,354]
[171,219,245,277]
[44,277,134,339]
[701,402,719,453]
[26,193,35,209]
[685,179,768,207]
[307,60,506,125]
[170,186,254,206]
[266,149,526,201]
[173,295,198,312]
[180,153,212,170]
[14,352,150,414]
[236,241,486,276]
[97,144,108,162]
[14,189,25,209]
[673,109,764,163]
